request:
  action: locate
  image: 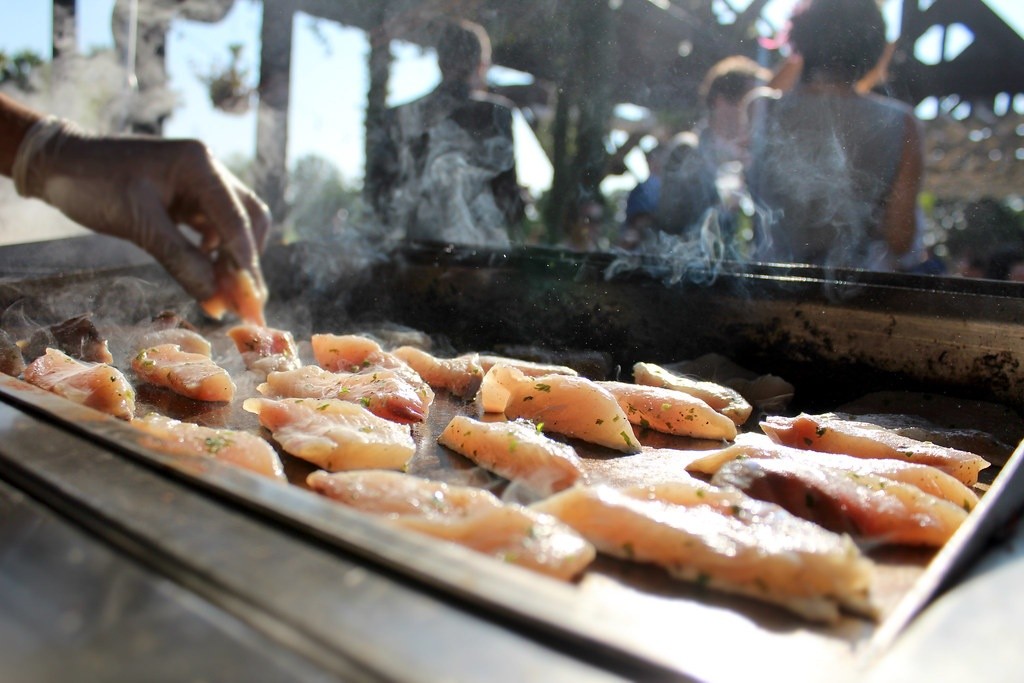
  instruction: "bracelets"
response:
[12,116,70,198]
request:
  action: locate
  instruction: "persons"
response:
[365,0,1024,282]
[0,93,272,306]
